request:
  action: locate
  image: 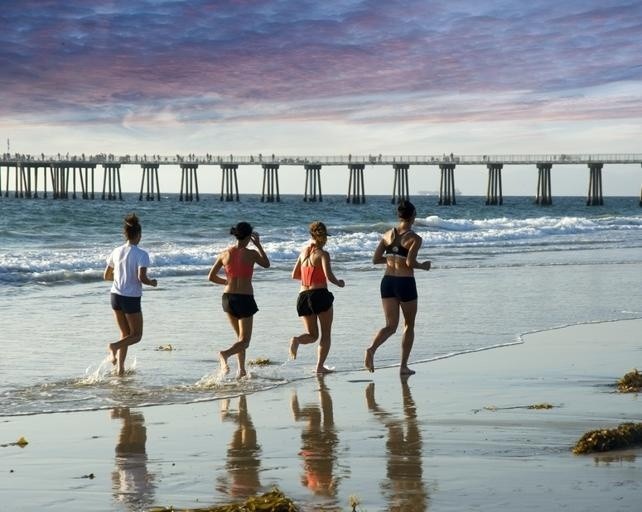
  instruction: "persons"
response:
[364,200,431,374]
[365,374,428,512]
[217,396,261,501]
[102,212,157,375]
[110,407,153,512]
[207,221,270,379]
[290,221,345,374]
[291,376,342,498]
